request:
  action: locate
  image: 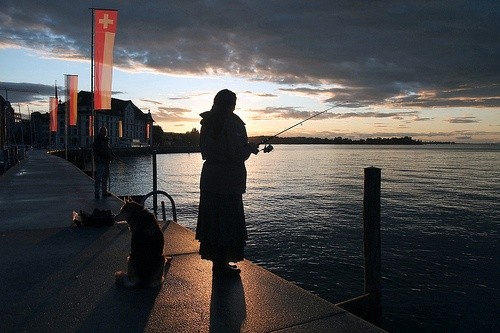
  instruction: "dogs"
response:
[114,197,167,296]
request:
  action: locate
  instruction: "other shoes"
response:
[102,192,111,198]
[211,262,241,277]
[95,193,100,199]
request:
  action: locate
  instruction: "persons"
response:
[194,89,260,275]
[92,127,111,200]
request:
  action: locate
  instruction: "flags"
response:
[89,116,94,136]
[147,124,149,138]
[90,9,118,111]
[119,121,122,137]
[65,75,79,126]
[50,97,58,132]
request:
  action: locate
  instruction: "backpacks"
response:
[74,208,116,227]
